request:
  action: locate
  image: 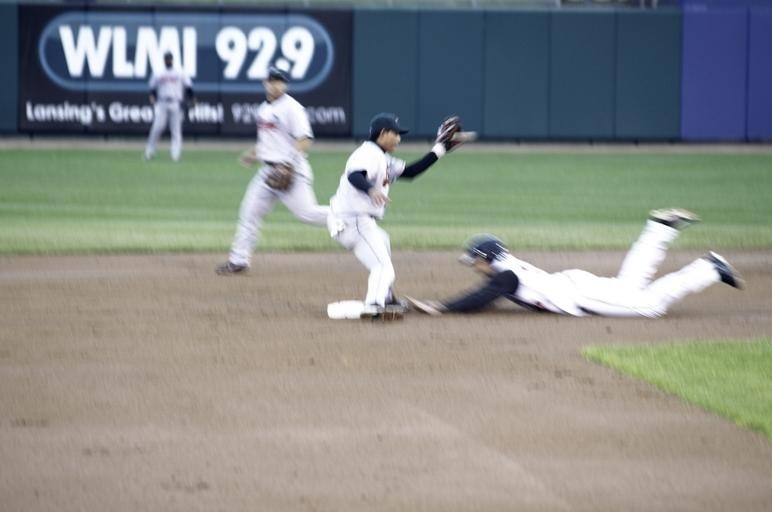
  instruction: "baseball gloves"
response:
[435,117,464,151]
[266,161,291,190]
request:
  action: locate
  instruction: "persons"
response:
[216,67,330,275]
[404,207,747,319]
[325,113,464,322]
[146,51,197,163]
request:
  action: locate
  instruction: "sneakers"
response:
[359,294,411,323]
[214,261,250,276]
[702,248,746,290]
[647,207,703,231]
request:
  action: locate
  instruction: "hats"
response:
[268,67,292,83]
[455,231,506,268]
[370,112,409,135]
